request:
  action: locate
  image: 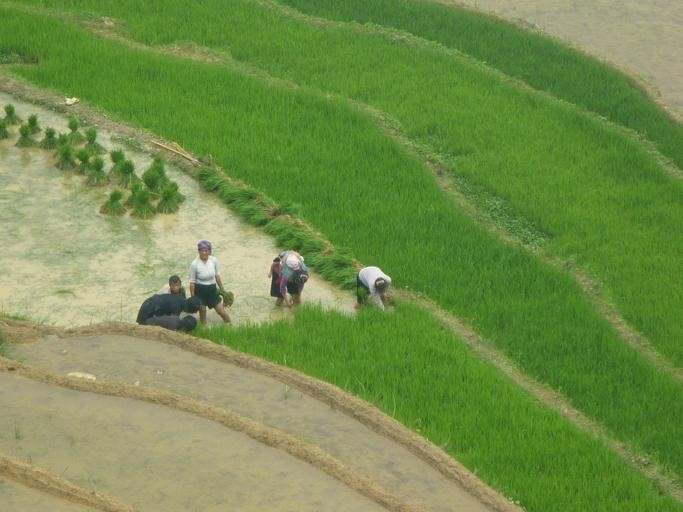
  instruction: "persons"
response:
[134,294,201,323]
[353,266,391,312]
[141,314,197,332]
[188,239,231,325]
[268,250,308,312]
[168,275,186,317]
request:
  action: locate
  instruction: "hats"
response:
[286,256,300,270]
